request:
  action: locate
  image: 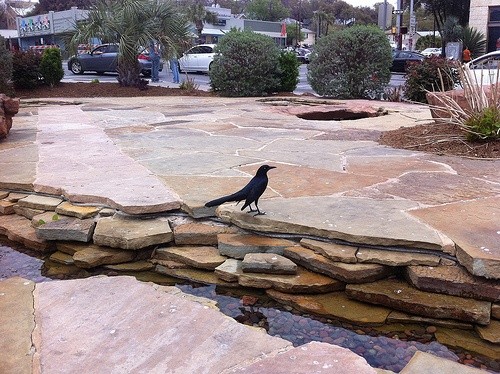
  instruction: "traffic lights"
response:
[401,26,408,35]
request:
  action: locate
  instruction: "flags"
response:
[281,23,286,36]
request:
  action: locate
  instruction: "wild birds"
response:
[202,159,281,220]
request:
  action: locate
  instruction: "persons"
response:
[463,46,471,69]
[169,45,179,83]
[147,30,162,82]
[496,37,500,60]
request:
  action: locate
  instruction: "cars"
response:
[389,51,431,74]
[454,51,500,90]
[285,47,317,64]
[68,42,164,78]
[173,44,221,74]
[390,41,406,51]
[421,48,442,59]
